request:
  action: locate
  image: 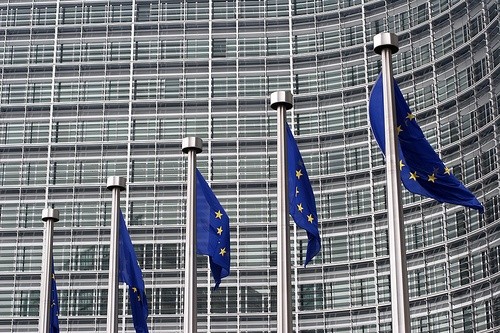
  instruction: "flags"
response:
[285,121,322,268]
[118,206,149,333]
[194,168,231,292]
[49,249,61,333]
[368,70,485,215]
